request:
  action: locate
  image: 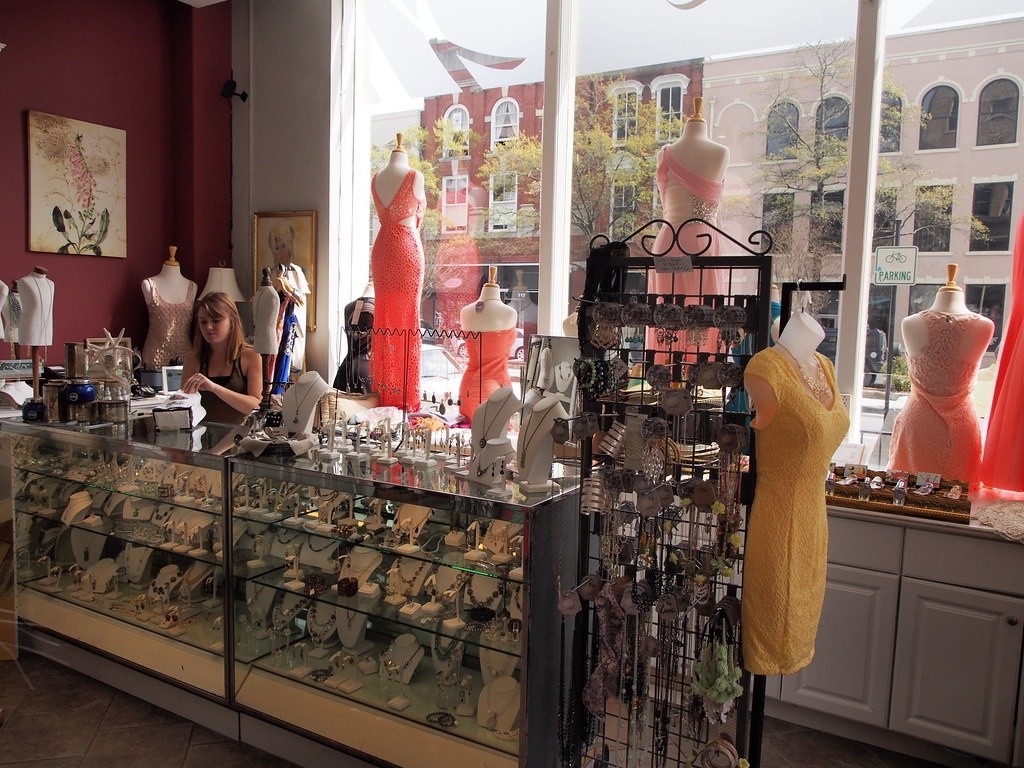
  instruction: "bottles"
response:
[62,379,95,404]
[21,397,48,423]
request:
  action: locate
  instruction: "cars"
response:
[453,328,524,360]
[419,341,466,398]
[420,318,438,345]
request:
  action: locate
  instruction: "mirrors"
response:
[519,334,580,425]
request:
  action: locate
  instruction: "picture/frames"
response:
[251,211,320,331]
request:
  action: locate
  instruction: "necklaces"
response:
[292,375,321,425]
[519,402,559,468]
[776,341,829,403]
[479,396,511,448]
[477,450,514,477]
[475,298,501,312]
[31,274,53,325]
[12,435,521,742]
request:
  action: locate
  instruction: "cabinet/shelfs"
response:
[620,492,1024,768]
[0,419,606,768]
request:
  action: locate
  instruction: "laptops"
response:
[162,365,183,395]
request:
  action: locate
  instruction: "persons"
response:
[252,281,278,354]
[474,386,523,458]
[370,150,427,414]
[516,397,568,484]
[334,284,375,395]
[885,286,994,485]
[180,291,263,424]
[459,283,517,423]
[141,260,198,372]
[649,117,730,388]
[17,266,55,346]
[282,371,335,435]
[468,437,517,486]
[742,309,851,673]
[2,286,23,342]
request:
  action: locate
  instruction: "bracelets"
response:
[573,355,615,401]
[556,419,744,768]
